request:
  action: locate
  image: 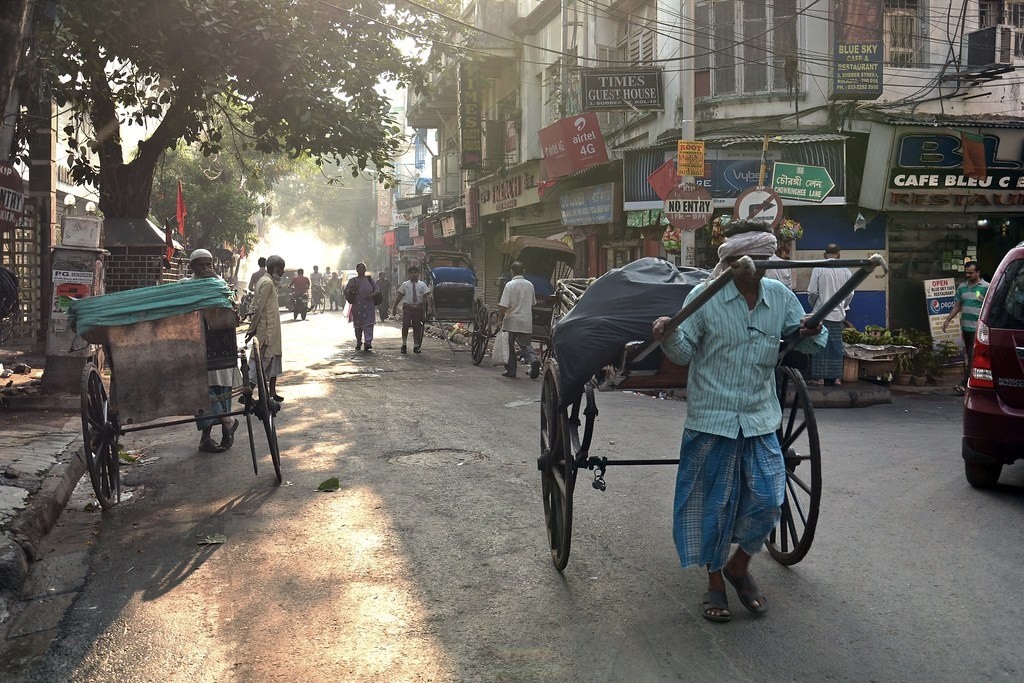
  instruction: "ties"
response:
[413,282,417,301]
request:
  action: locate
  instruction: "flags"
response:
[176,184,187,236]
[166,222,174,260]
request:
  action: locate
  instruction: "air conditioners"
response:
[966,24,1014,68]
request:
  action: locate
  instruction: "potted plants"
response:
[841,325,958,386]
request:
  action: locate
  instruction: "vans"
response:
[958,244,1024,489]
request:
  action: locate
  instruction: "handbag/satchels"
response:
[492,328,509,367]
[367,276,383,306]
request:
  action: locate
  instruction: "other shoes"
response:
[401,345,407,353]
[502,370,517,376]
[356,341,362,350]
[364,344,372,350]
[413,347,422,353]
[530,360,541,380]
[238,395,255,405]
[270,394,284,402]
[806,379,824,388]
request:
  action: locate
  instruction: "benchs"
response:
[432,268,476,294]
[502,272,560,306]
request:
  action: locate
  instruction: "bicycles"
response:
[311,283,328,313]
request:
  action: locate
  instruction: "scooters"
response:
[288,285,311,321]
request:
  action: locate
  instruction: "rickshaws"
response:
[536,248,887,575]
[65,274,285,510]
[492,235,579,360]
[420,249,487,358]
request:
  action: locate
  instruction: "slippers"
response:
[220,419,239,449]
[723,567,769,613]
[700,590,731,622]
[952,383,965,395]
[199,439,226,452]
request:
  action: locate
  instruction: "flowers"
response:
[711,214,733,245]
[662,226,682,250]
[772,218,805,258]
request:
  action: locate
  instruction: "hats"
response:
[190,248,213,259]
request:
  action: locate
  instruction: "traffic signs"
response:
[770,161,840,203]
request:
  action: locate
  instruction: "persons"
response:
[377,272,391,322]
[654,219,828,621]
[807,245,855,387]
[345,263,381,349]
[249,257,266,291]
[238,256,285,402]
[288,267,340,312]
[941,261,989,393]
[765,254,791,291]
[496,262,541,378]
[178,249,240,453]
[393,266,431,353]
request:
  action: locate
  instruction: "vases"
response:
[665,249,679,254]
